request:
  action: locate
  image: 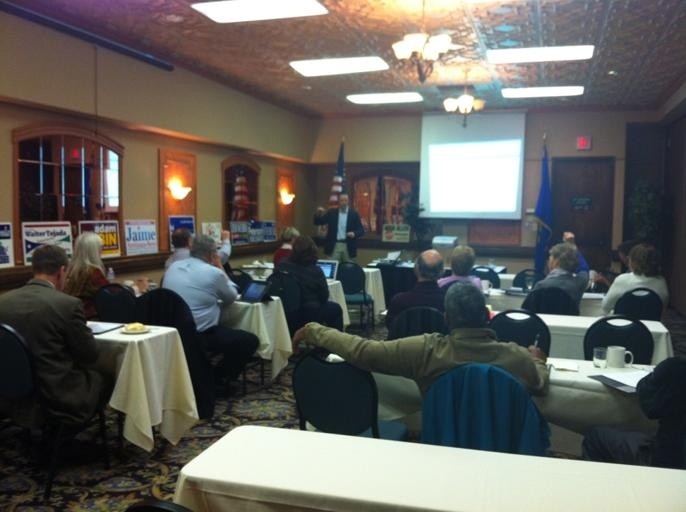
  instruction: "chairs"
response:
[89,261,661,367]
[2,323,122,498]
[292,345,413,440]
[137,288,247,420]
[422,361,550,456]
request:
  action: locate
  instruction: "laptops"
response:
[315,259,338,282]
[239,280,273,303]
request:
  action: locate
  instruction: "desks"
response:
[324,332,663,459]
[176,423,686,512]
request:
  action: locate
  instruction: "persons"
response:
[161,233,261,402]
[312,192,365,264]
[273,225,302,270]
[293,279,550,398]
[58,229,152,323]
[600,241,671,320]
[534,231,591,310]
[386,247,447,328]
[437,244,482,291]
[591,237,638,288]
[278,232,344,333]
[1,242,117,465]
[163,227,232,271]
[579,353,686,469]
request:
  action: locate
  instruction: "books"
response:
[85,318,123,335]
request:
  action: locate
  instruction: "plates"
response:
[122,328,152,335]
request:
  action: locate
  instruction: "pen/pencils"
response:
[534,332,541,347]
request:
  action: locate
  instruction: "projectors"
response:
[432,235,459,249]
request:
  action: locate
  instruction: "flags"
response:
[326,134,347,210]
[532,128,554,273]
[229,164,254,221]
[372,173,387,234]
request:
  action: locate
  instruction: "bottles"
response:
[106,267,115,284]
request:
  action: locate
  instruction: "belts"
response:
[336,240,346,243]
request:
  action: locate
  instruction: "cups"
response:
[608,346,634,369]
[481,280,494,291]
[593,347,608,369]
